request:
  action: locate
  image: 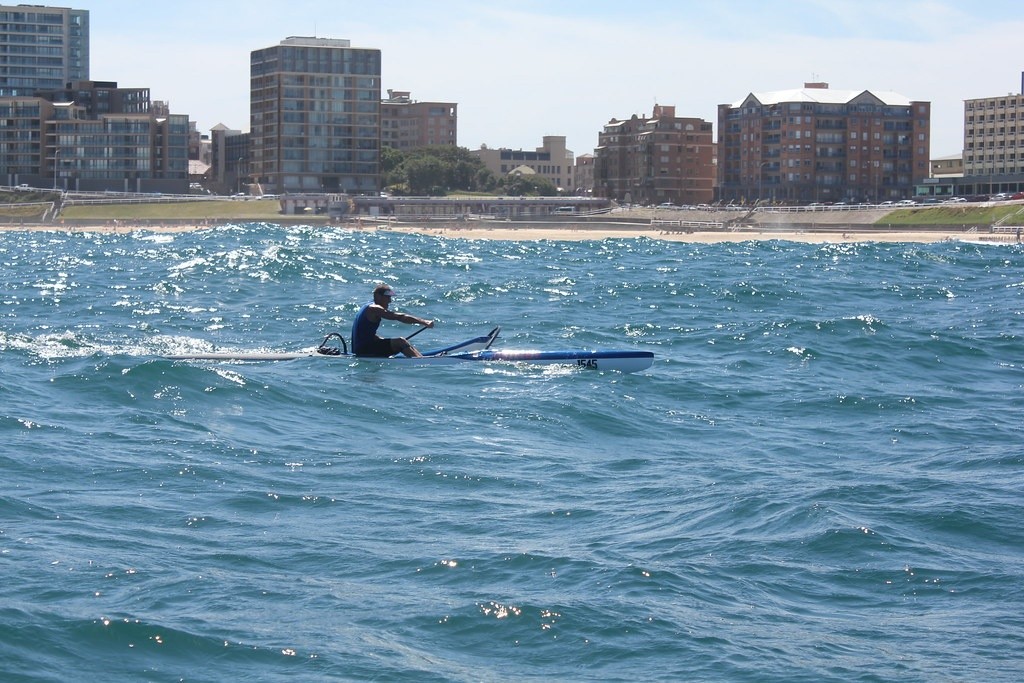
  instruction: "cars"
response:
[614,190,1023,212]
[380,191,388,198]
[190,182,280,201]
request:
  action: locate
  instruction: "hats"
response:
[372,283,397,297]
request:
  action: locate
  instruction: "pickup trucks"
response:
[14,183,34,192]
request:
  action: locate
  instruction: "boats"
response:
[161,348,654,374]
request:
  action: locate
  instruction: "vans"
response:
[550,207,576,215]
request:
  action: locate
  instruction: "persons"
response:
[350,283,435,359]
